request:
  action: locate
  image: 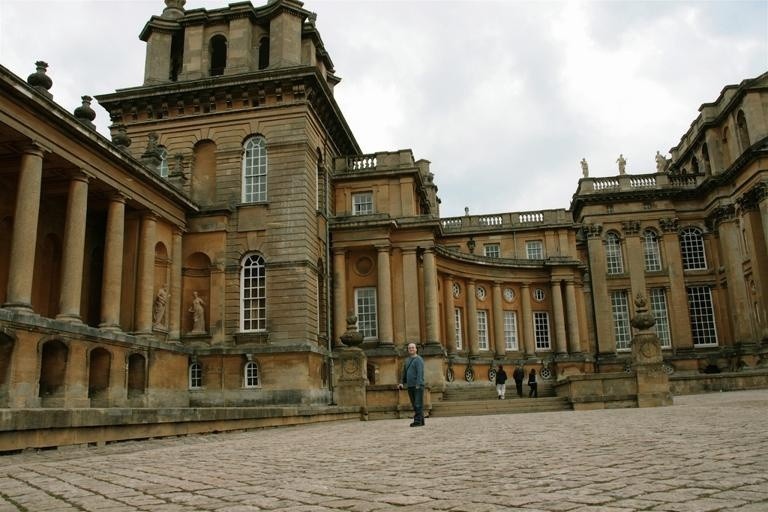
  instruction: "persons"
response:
[615,154,626,173]
[580,157,589,177]
[152,283,171,324]
[396,341,426,427]
[188,290,207,331]
[513,363,524,398]
[527,368,538,397]
[654,150,667,169]
[492,363,507,400]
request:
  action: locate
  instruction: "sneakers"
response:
[408,421,426,426]
